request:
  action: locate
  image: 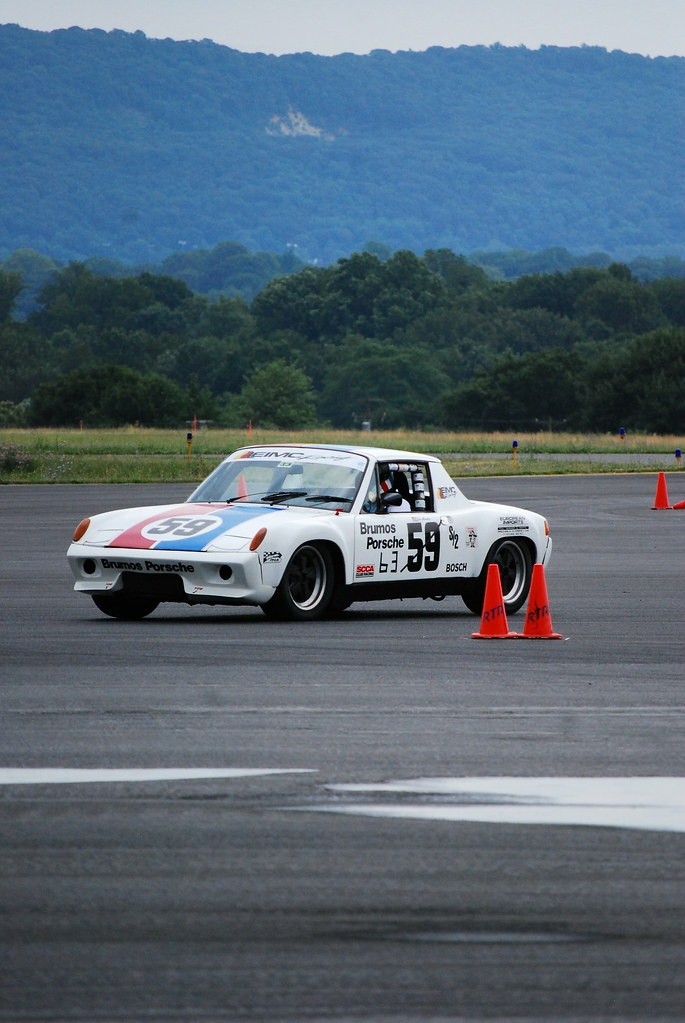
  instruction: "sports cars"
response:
[68,444,553,624]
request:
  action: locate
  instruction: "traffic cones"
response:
[649,472,674,511]
[469,562,524,638]
[515,561,564,640]
[237,473,248,503]
[673,499,685,509]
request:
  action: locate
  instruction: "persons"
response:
[309,469,411,512]
